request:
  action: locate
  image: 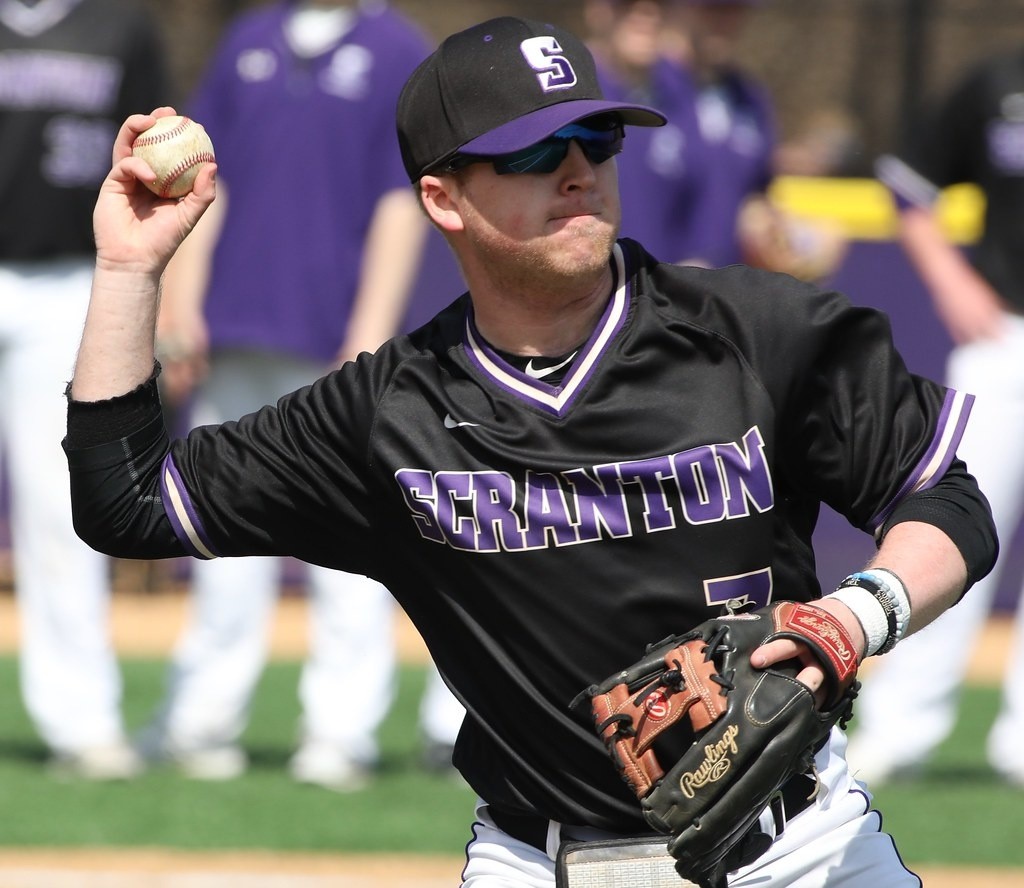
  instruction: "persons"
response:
[1,0,182,785]
[61,14,1001,888]
[654,2,855,281]
[837,41,1024,794]
[150,0,439,795]
[578,1,739,276]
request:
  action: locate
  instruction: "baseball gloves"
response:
[567,599,862,888]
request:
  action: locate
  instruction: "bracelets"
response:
[835,577,897,656]
[841,572,904,654]
[833,581,894,657]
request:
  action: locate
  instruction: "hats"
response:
[396,16,668,185]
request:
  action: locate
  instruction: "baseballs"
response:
[132,115,216,200]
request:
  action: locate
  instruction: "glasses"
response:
[433,118,623,177]
